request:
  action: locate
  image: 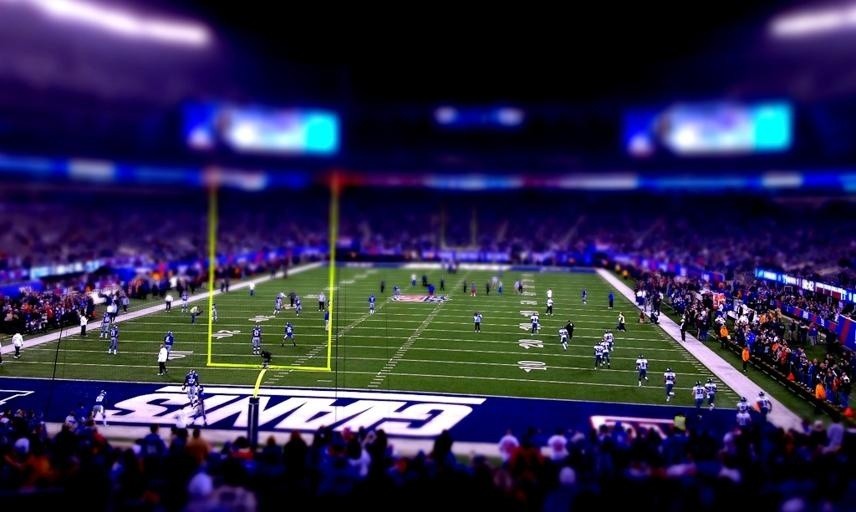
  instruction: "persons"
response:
[1,211,855,511]
